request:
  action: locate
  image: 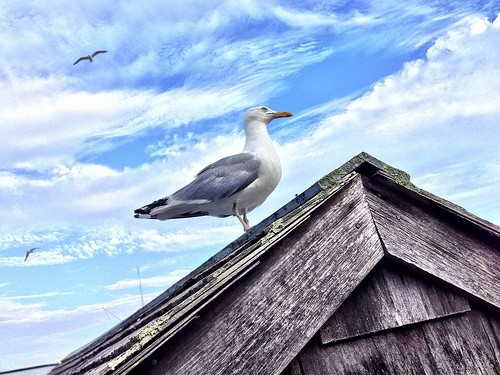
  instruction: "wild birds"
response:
[23,247,38,262]
[133,106,294,233]
[72,50,108,65]
